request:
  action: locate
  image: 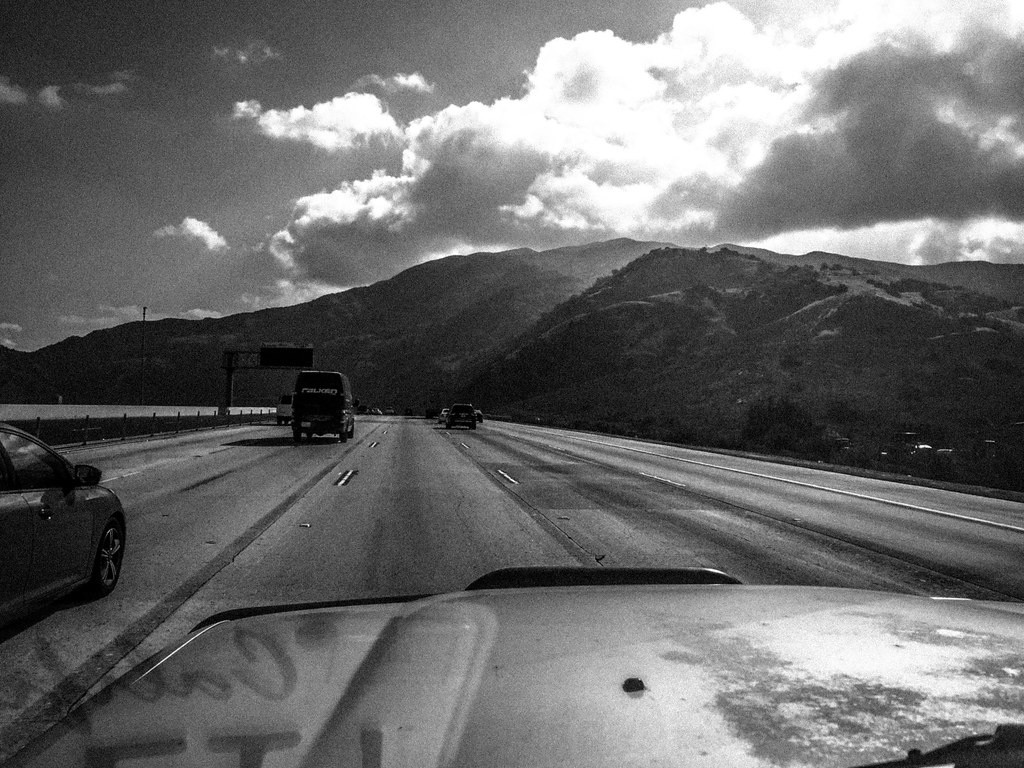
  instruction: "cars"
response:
[384,406,396,416]
[356,404,383,416]
[0,424,129,615]
[474,409,484,423]
[438,408,450,424]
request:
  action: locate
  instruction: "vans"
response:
[291,370,361,443]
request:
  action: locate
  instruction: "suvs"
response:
[275,392,294,426]
[445,403,477,430]
